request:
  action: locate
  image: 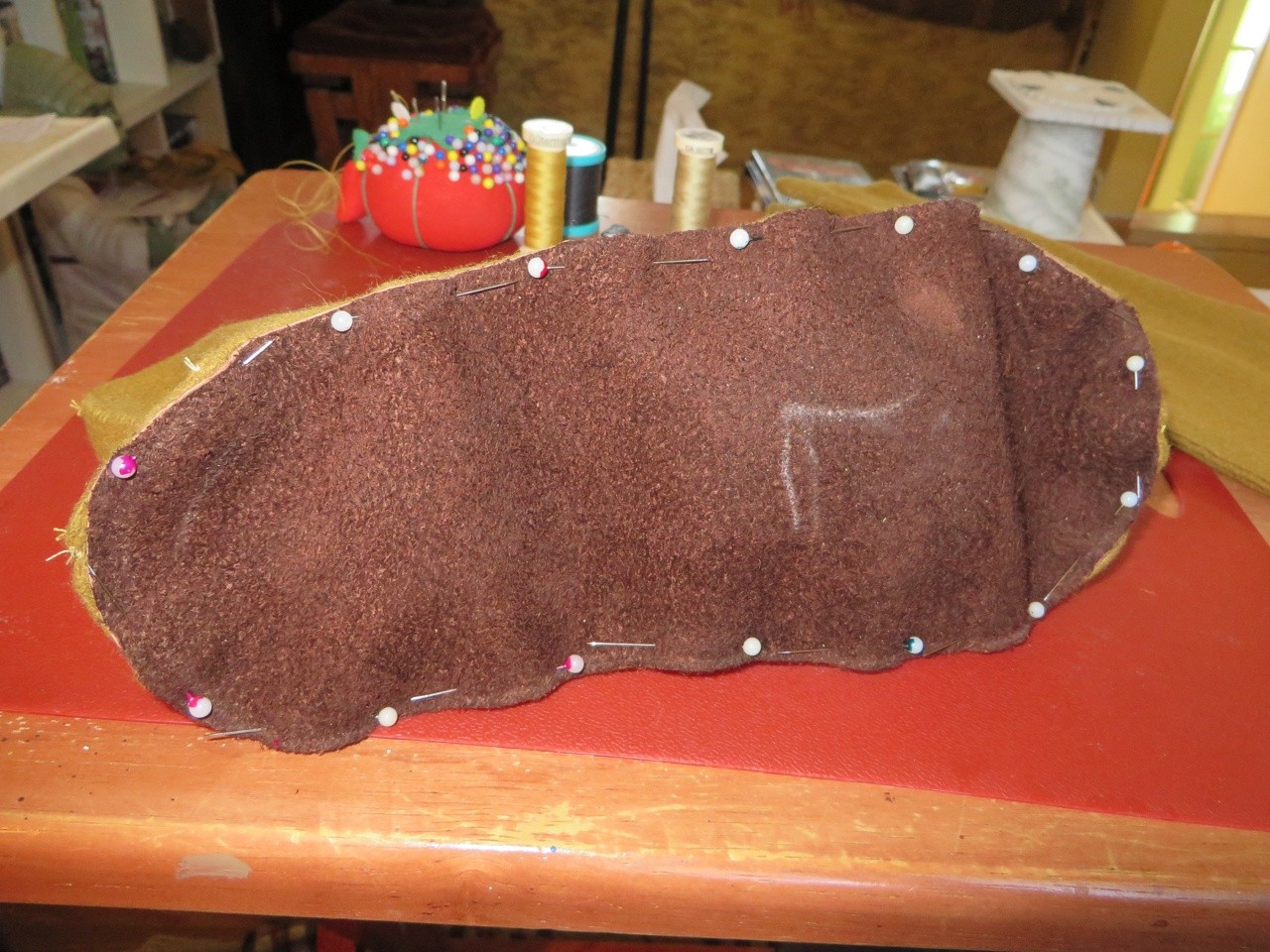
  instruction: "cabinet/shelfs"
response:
[0,0,239,350]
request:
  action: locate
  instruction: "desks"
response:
[0,171,1270,952]
[0,118,120,423]
